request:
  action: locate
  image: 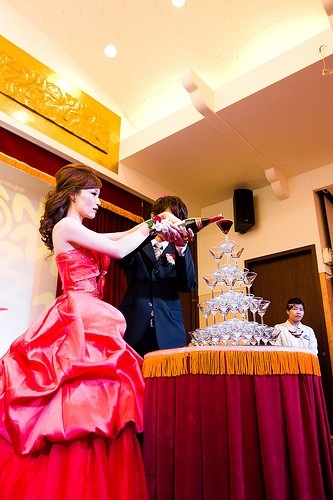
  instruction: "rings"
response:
[184,237,188,241]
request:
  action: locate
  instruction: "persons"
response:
[111,195,197,449]
[273,298,319,353]
[0,163,184,500]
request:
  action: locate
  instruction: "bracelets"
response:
[146,215,170,237]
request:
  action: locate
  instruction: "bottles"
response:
[158,212,224,242]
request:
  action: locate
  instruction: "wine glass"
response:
[215,218,233,244]
[189,242,281,347]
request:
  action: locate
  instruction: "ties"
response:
[153,243,162,261]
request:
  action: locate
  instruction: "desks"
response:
[140,345,333,500]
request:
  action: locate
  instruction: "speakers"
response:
[232,188,255,234]
